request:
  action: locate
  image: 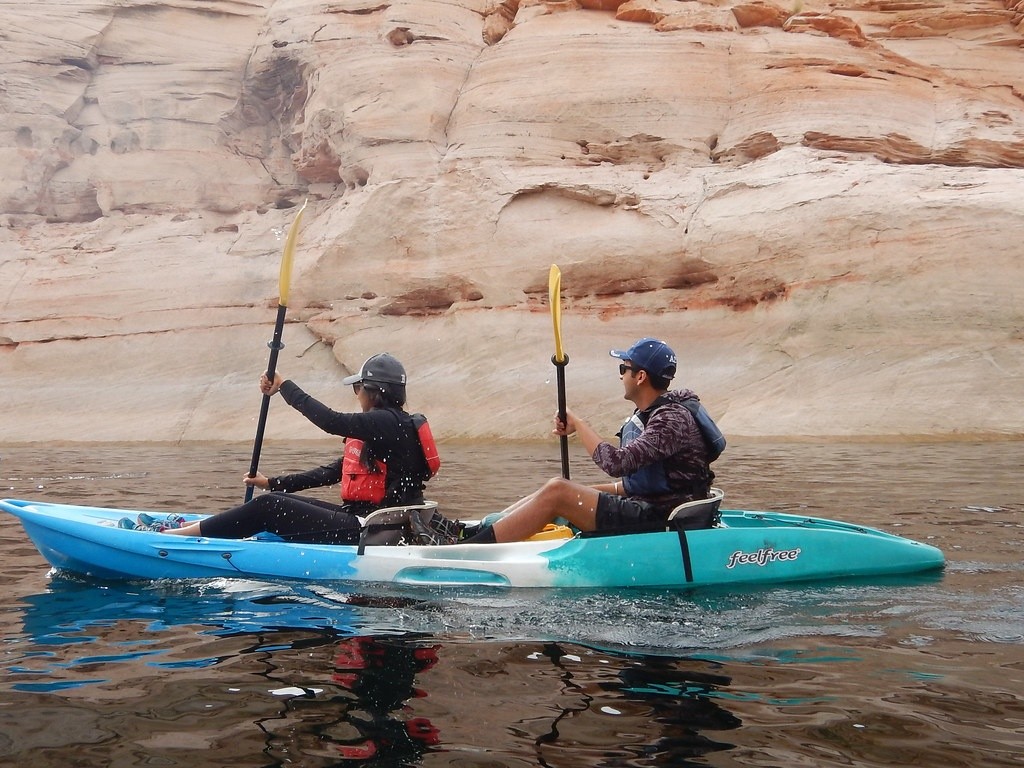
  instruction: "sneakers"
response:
[410,510,459,545]
[118,518,164,533]
[430,509,466,540]
[137,512,186,530]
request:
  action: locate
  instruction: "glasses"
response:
[352,382,364,395]
[620,364,648,375]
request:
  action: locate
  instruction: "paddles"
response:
[242,199,307,498]
[546,263,572,480]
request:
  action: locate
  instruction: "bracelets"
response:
[615,483,617,495]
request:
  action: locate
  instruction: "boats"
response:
[0,498,945,595]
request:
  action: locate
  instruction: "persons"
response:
[119,350,440,545]
[409,337,727,546]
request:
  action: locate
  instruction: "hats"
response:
[343,353,407,386]
[609,338,677,380]
